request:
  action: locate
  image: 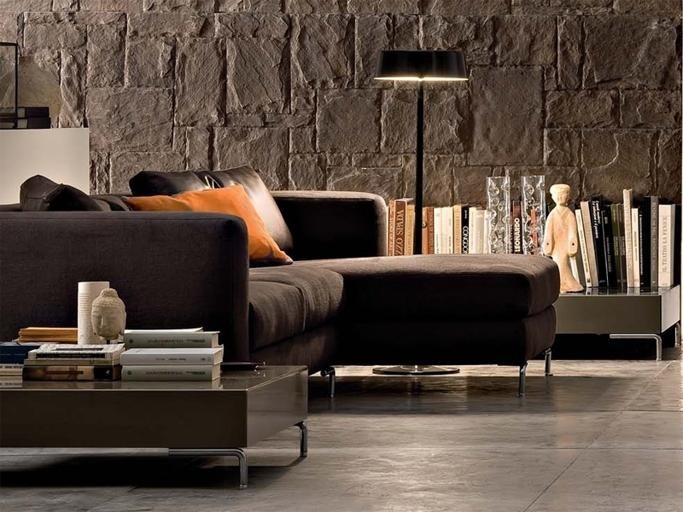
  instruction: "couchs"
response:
[0,165,559,412]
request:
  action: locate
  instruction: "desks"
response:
[549,282,680,360]
[0,363,311,491]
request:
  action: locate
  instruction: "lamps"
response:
[374,49,468,375]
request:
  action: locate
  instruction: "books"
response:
[382,184,676,291]
[0,280,225,383]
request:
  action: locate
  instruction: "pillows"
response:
[119,183,295,268]
